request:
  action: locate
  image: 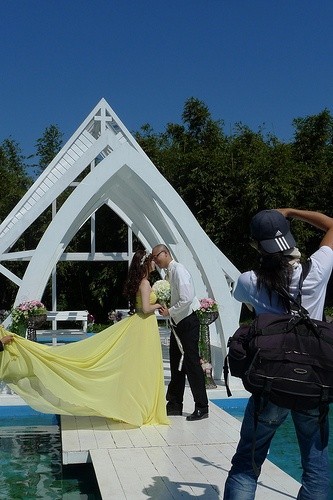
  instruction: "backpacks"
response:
[243,259,333,407]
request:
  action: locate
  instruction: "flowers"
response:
[87,314,95,333]
[108,311,122,323]
[195,299,218,311]
[152,280,177,328]
[14,301,46,326]
[199,356,212,378]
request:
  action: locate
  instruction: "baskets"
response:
[196,312,219,390]
[20,314,47,342]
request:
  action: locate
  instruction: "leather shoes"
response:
[186,411,208,421]
[167,409,182,416]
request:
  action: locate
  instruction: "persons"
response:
[0,334,14,351]
[223,207,333,500]
[0,249,172,428]
[150,243,209,422]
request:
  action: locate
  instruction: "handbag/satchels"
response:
[223,302,256,397]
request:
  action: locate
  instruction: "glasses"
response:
[152,251,164,261]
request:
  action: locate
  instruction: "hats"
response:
[250,209,296,254]
